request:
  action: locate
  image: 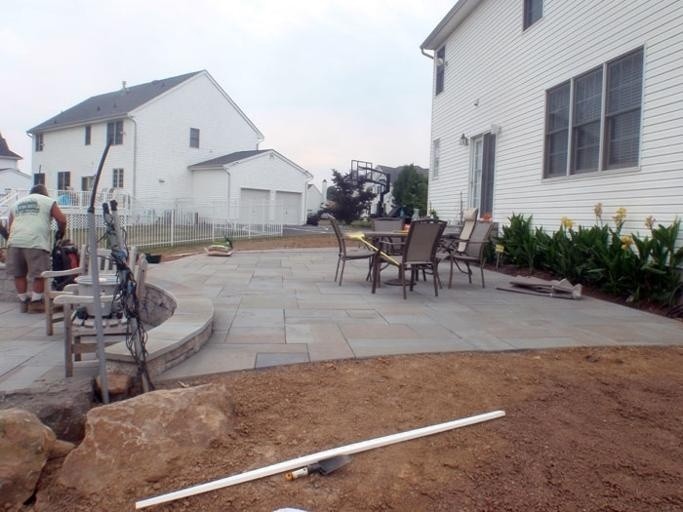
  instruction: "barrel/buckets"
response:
[75,274,122,318]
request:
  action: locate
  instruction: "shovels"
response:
[285,455,353,481]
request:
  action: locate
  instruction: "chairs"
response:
[39,244,149,378]
[326,206,497,299]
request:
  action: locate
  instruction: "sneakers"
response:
[17,298,45,314]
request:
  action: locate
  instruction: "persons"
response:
[6,184,66,313]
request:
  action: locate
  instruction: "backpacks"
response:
[52,239,79,291]
